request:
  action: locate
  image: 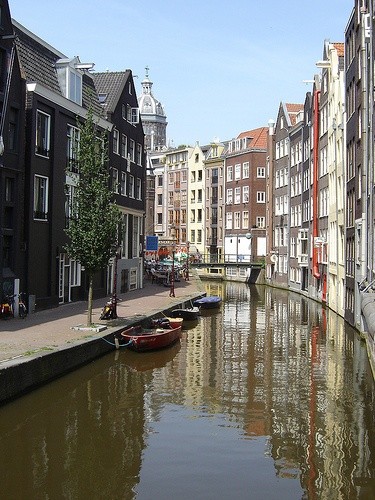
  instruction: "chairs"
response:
[148,270,168,286]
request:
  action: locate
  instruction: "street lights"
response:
[112,245,122,319]
[168,242,177,298]
[185,240,191,281]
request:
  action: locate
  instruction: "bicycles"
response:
[2,295,17,322]
[98,293,121,321]
[17,291,28,320]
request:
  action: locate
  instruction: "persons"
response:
[144,257,158,275]
[185,269,189,281]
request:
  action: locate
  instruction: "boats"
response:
[171,306,201,320]
[120,325,182,353]
[152,316,184,329]
[193,295,222,310]
